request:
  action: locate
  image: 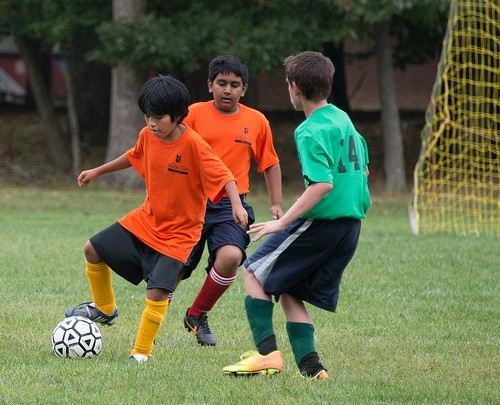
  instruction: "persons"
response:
[178,55,285,347]
[221,51,371,379]
[64,77,249,365]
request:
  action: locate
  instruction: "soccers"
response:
[51,316,102,360]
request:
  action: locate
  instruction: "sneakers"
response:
[223,350,283,377]
[296,370,328,382]
[65,302,119,327]
[129,354,149,362]
[183,307,217,347]
